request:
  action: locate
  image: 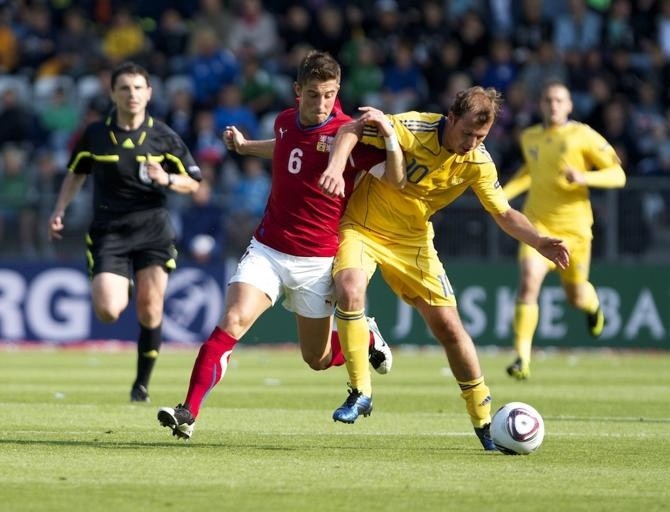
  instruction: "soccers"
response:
[490,402,544,455]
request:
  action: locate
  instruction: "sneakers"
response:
[158,407,194,438]
[131,383,149,402]
[333,390,373,423]
[507,360,530,380]
[588,302,604,336]
[367,317,392,374]
[475,423,496,450]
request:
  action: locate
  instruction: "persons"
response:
[0,1,669,269]
[156,52,409,442]
[317,85,573,452]
[48,63,212,402]
[495,80,629,378]
